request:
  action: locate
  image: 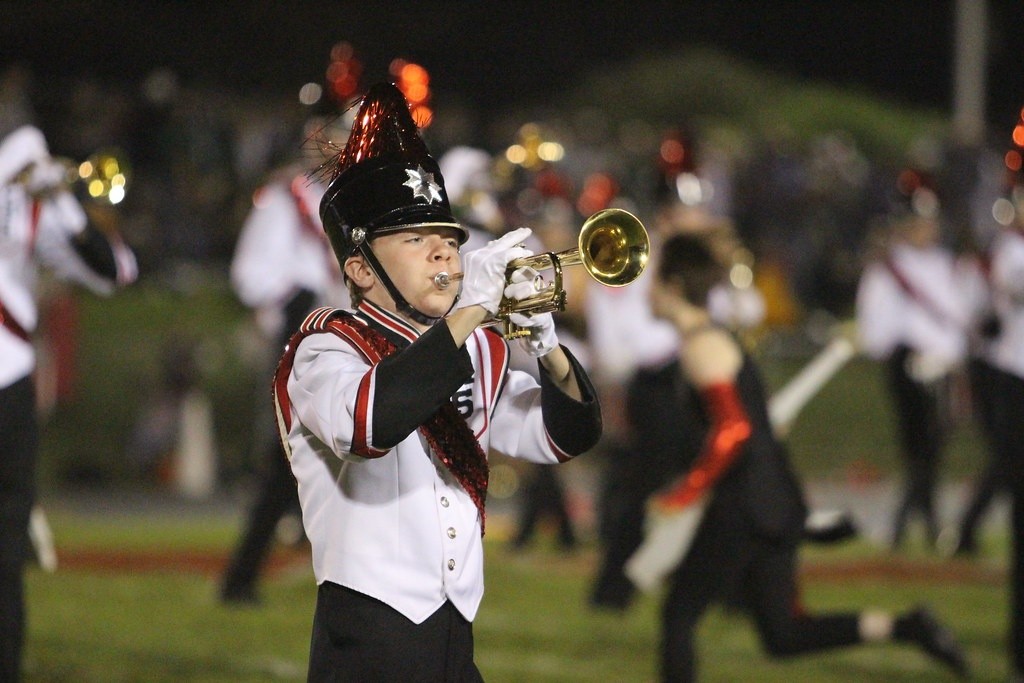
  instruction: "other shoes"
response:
[509,533,530,549]
[221,580,258,606]
[593,571,634,608]
[959,536,977,558]
[555,529,575,546]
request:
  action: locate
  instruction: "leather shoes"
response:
[894,602,971,683]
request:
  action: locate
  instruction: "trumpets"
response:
[431,207,652,342]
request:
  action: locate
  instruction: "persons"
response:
[0,125,137,683]
[219,94,358,606]
[855,108,1024,561]
[271,81,603,683]
[647,232,975,683]
[436,121,767,617]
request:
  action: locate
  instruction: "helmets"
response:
[319,153,469,285]
